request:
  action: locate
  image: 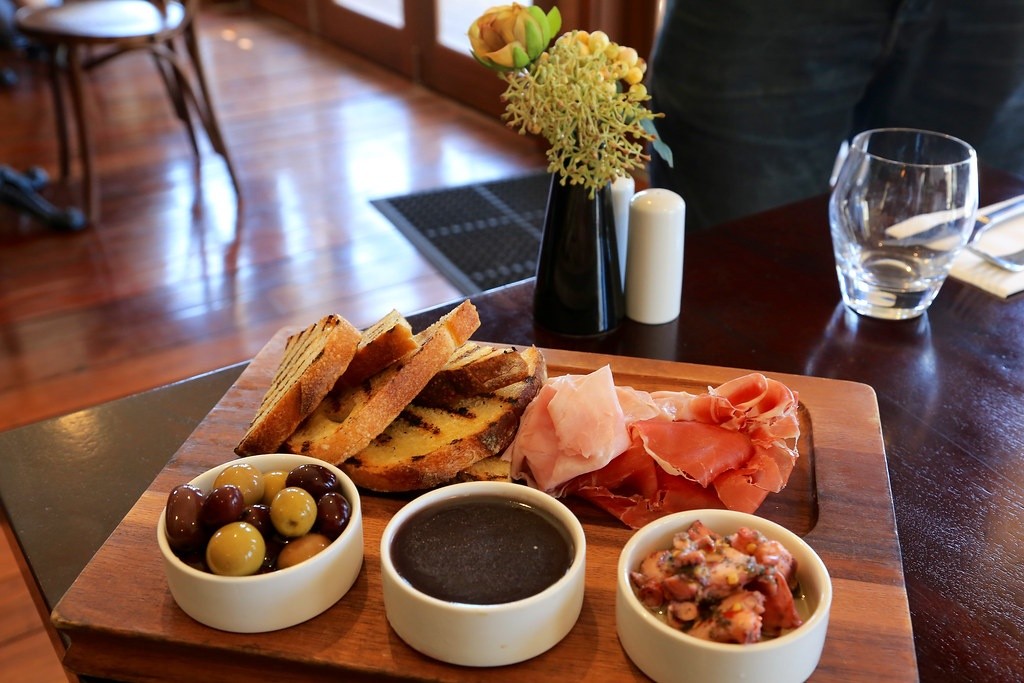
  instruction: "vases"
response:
[531,146,624,338]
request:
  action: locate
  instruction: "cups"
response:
[830,127,979,320]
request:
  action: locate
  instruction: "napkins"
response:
[882,198,1024,300]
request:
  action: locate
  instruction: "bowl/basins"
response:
[156,453,362,633]
[616,509,831,682]
[380,481,586,668]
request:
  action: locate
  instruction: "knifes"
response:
[903,200,1024,244]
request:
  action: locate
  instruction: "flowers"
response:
[468,3,666,199]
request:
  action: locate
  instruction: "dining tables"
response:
[1,160,1023,683]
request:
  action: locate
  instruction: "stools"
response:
[14,0,242,224]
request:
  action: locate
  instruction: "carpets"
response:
[368,171,559,298]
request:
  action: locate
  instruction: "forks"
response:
[966,245,1024,271]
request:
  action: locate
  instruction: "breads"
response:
[232,298,549,494]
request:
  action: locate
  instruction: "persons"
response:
[645,0,1024,233]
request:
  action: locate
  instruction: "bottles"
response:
[608,169,685,325]
